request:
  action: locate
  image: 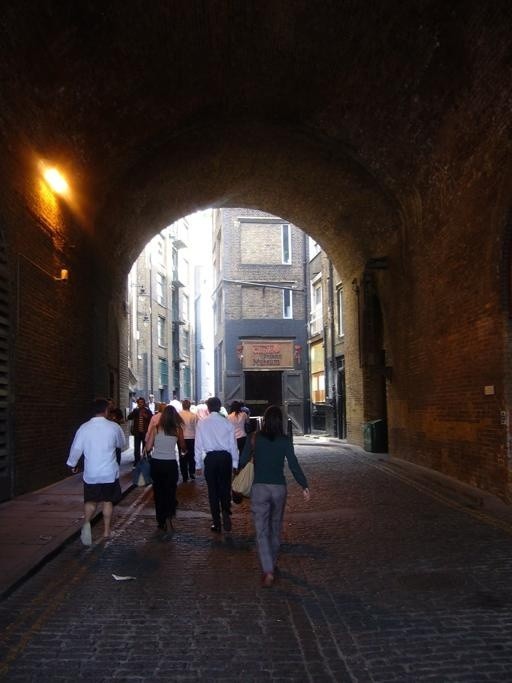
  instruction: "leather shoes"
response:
[211,525,231,531]
[80,521,92,546]
[103,531,116,539]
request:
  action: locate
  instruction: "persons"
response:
[127,395,199,532]
[194,396,252,536]
[239,406,311,587]
[67,397,128,549]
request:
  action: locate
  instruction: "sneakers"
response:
[157,520,174,535]
[263,572,273,588]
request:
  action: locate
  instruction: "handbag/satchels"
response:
[232,431,257,497]
[133,447,152,486]
